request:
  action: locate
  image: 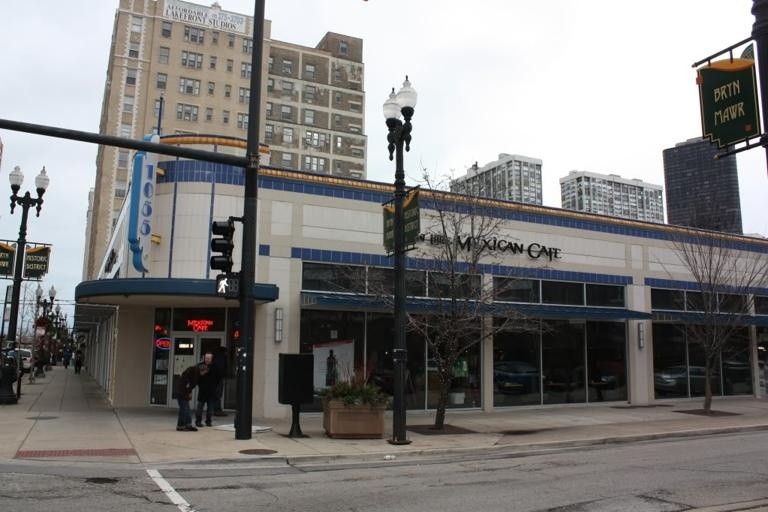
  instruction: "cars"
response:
[0,348,32,384]
[493,356,627,395]
[654,359,753,399]
[367,352,481,397]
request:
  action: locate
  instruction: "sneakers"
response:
[186,426,197,431]
[178,428,192,431]
[214,411,224,415]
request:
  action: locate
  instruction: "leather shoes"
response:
[195,420,201,426]
[205,421,211,426]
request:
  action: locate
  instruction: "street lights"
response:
[4,165,50,349]
[33,283,68,372]
[381,74,418,447]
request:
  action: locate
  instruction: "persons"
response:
[196,352,218,427]
[52,348,83,374]
[175,364,208,431]
[213,347,228,416]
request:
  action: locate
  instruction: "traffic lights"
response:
[209,215,237,276]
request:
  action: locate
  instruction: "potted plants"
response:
[322,369,389,438]
